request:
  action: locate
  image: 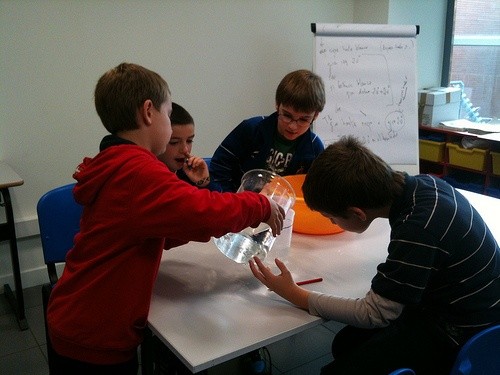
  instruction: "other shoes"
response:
[320,360,339,375]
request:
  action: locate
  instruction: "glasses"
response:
[278,112,313,128]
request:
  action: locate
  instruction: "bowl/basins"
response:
[267,174,346,235]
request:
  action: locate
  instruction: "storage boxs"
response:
[418,136,500,199]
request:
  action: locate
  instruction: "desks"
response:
[0,160,29,331]
[146,186,500,375]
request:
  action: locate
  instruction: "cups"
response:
[273,209,294,249]
[212,168,296,265]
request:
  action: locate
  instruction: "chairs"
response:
[388,325,500,375]
[36,182,83,310]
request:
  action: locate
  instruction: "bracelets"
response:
[196,176,210,186]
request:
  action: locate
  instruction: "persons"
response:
[47,62,287,375]
[158,101,210,193]
[206,68,326,193]
[247,137,500,375]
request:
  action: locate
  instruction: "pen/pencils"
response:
[268,278,324,291]
